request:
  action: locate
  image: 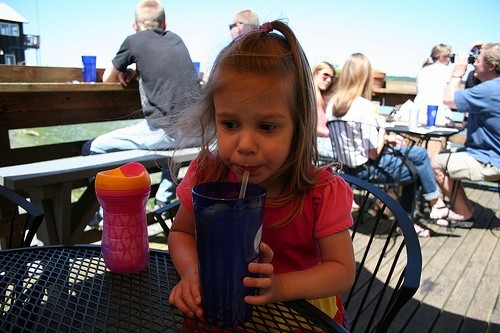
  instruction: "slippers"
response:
[436,218,474,228]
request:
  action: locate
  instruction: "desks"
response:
[0,242,350,332]
[0,62,194,244]
[385,123,465,152]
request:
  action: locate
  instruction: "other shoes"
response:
[87,213,104,232]
[154,205,168,220]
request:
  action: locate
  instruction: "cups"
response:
[81,55,96,83]
[96,163,152,274]
[192,62,200,83]
[192,182,266,327]
[427,105,438,127]
[408,107,420,129]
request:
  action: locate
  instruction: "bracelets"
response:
[380,126,385,130]
[451,75,463,79]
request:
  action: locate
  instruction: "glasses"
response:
[228,22,243,30]
[322,72,338,83]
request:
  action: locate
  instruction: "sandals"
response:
[429,208,464,221]
[396,223,430,237]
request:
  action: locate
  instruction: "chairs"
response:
[326,119,424,231]
[153,169,423,333]
[0,183,46,250]
[447,171,500,228]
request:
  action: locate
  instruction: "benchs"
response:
[0,137,219,243]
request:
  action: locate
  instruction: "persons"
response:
[80,0,214,231]
[414,43,456,154]
[326,52,465,237]
[430,42,500,229]
[166,20,358,333]
[309,60,362,210]
[229,9,260,41]
[465,44,486,89]
